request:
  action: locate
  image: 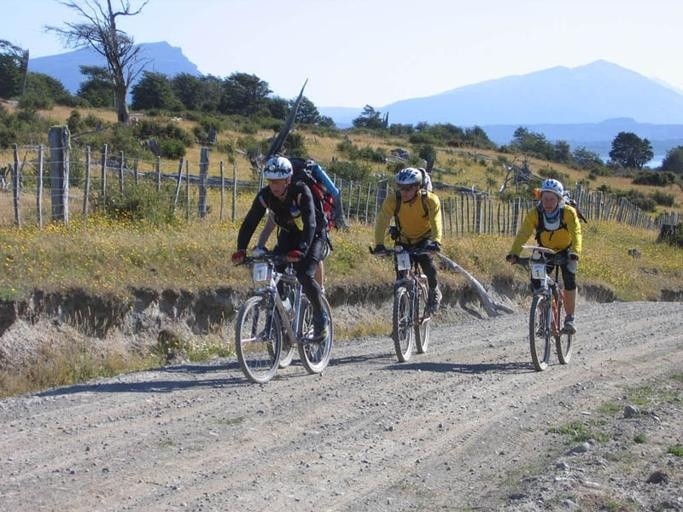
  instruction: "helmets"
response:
[540,180,563,196]
[395,167,422,186]
[263,155,294,180]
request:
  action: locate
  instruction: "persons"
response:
[257,209,325,296]
[229,155,329,345]
[504,178,584,335]
[372,168,442,319]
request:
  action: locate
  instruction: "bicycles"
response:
[511,245,575,371]
[234,254,333,382]
[367,245,433,361]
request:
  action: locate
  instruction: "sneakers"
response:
[560,321,576,335]
[312,311,329,334]
[428,286,442,311]
